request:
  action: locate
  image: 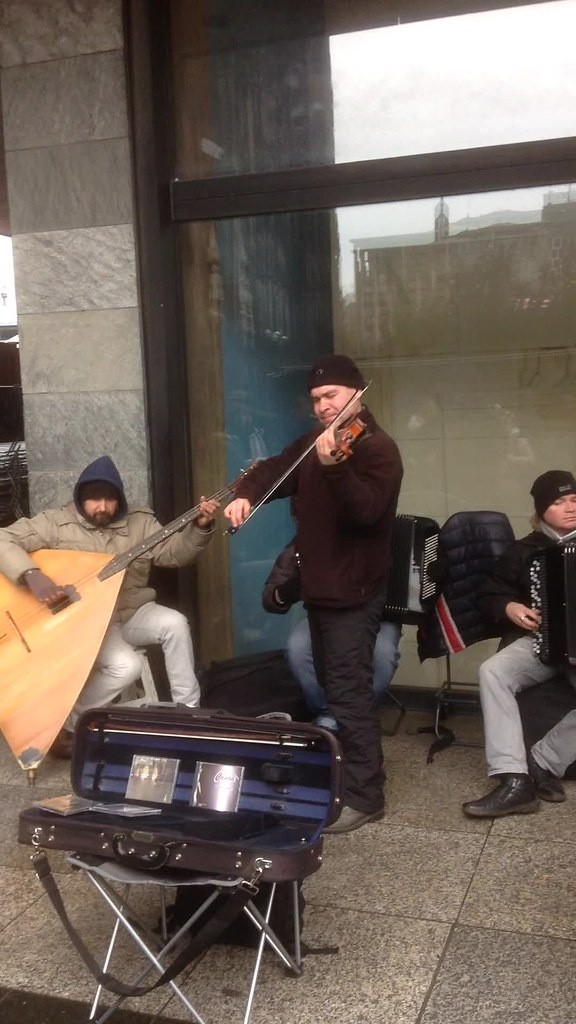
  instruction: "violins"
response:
[329,415,369,464]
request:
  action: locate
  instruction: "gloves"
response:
[277,584,300,603]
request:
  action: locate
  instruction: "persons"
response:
[0,456,222,759]
[224,354,403,834]
[262,534,402,734]
[461,469,576,817]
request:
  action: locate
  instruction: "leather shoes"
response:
[321,804,385,833]
[462,773,538,817]
[528,755,566,802]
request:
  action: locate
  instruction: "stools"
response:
[65,852,304,1024]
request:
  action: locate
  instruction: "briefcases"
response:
[19,705,344,882]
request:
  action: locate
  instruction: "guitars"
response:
[0,458,265,789]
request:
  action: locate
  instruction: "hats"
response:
[308,354,364,394]
[530,470,576,516]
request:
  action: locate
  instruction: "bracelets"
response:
[20,570,34,585]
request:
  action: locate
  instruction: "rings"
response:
[520,616,527,622]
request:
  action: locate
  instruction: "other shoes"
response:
[51,727,74,758]
